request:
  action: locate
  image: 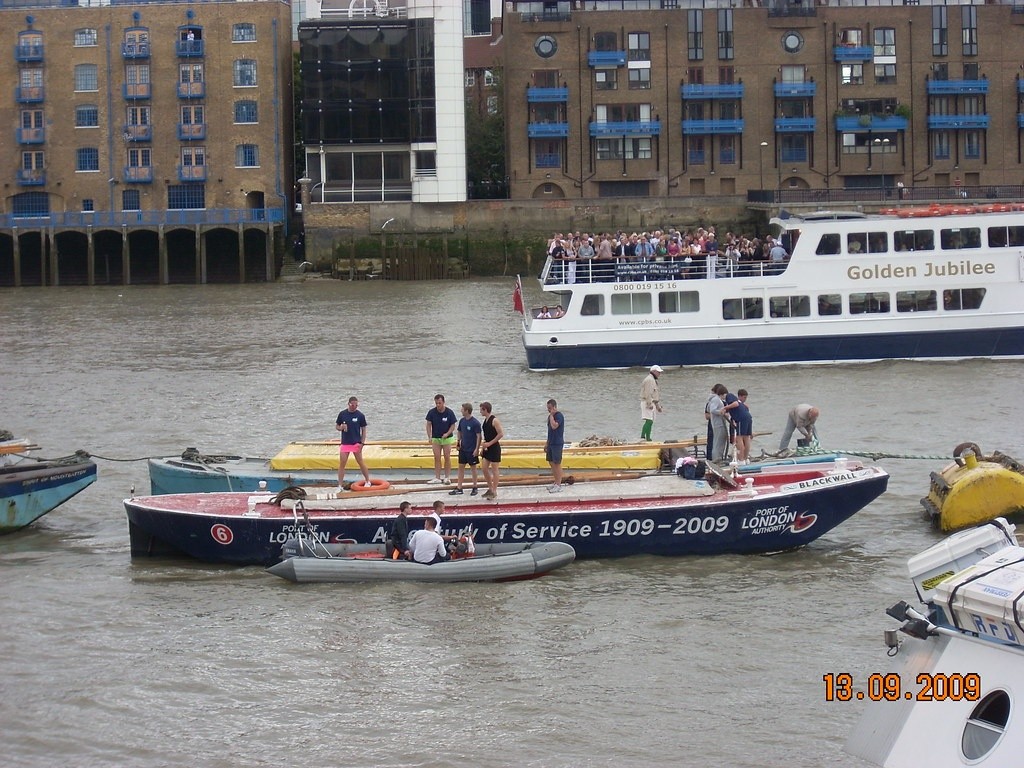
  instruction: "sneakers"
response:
[470,487,479,497]
[449,486,464,495]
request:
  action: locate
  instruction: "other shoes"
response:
[746,459,751,464]
[444,477,451,485]
[549,484,563,493]
[334,485,344,494]
[427,478,443,484]
[364,481,371,487]
[487,492,498,500]
[546,482,556,490]
[481,490,491,498]
[729,460,746,467]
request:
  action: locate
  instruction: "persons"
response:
[390,500,458,565]
[954,176,967,198]
[544,398,565,493]
[334,397,372,494]
[537,305,565,320]
[819,226,1024,256]
[639,365,663,442]
[704,384,754,466]
[898,180,903,200]
[425,394,504,501]
[722,288,983,321]
[186,28,196,52]
[778,404,818,456]
[545,226,790,285]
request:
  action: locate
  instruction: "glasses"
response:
[349,402,358,407]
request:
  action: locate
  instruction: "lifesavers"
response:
[880,202,1024,218]
[349,479,390,492]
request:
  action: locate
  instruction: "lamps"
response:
[884,599,940,657]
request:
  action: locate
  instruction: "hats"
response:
[649,365,664,374]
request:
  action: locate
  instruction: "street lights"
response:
[760,141,768,200]
[875,138,890,200]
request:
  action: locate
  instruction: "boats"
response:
[264,538,576,582]
[516,201,1024,372]
[150,436,711,496]
[0,428,97,535]
[840,514,1024,768]
[123,453,889,567]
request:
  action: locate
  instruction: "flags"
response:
[513,277,523,315]
